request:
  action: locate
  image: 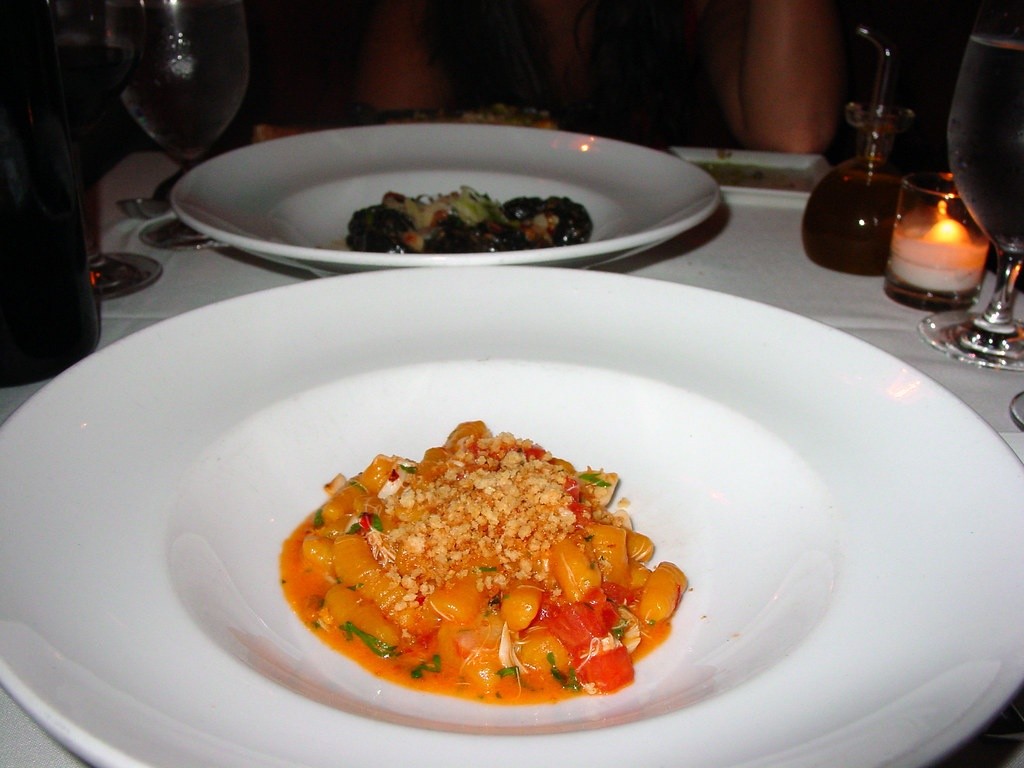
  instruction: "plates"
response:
[2,267,1022,768]
[172,122,720,279]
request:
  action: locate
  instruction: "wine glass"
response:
[918,34,1024,372]
[105,1,250,249]
[48,0,165,299]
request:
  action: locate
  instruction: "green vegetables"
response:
[314,459,660,696]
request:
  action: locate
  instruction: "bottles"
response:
[1,1,102,389]
[802,101,914,275]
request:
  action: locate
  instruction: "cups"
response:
[885,172,988,311]
